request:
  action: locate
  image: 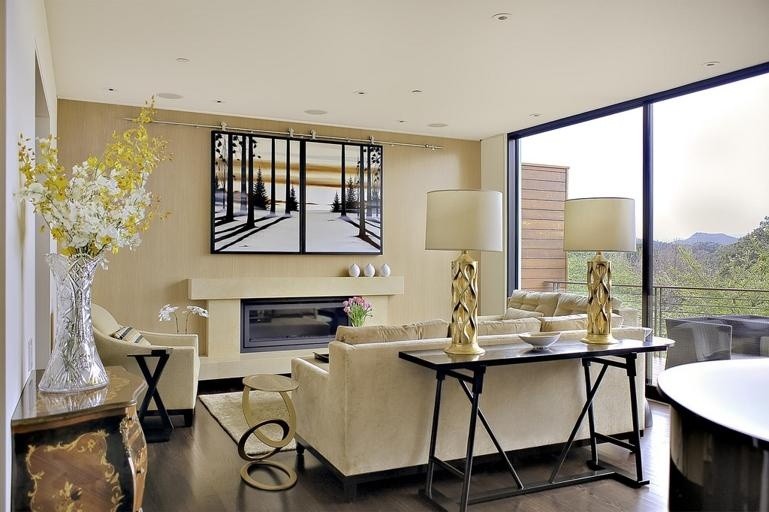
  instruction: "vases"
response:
[36,252,110,393]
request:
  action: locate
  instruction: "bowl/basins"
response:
[520,331,565,348]
[519,348,554,356]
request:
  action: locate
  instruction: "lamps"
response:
[563,197,636,344]
[425,188,503,355]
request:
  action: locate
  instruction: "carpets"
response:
[198,391,297,456]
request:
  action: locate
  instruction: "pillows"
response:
[334,290,623,346]
[111,325,152,346]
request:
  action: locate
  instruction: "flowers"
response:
[158,304,180,334]
[182,306,209,334]
[14,94,173,370]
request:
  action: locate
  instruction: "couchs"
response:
[292,289,646,500]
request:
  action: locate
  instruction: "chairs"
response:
[664,314,769,370]
[90,302,199,427]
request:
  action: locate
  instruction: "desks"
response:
[10,366,148,512]
[658,357,769,512]
[398,336,675,512]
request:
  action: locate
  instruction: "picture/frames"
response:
[210,130,383,256]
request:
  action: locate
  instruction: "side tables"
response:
[237,374,299,491]
[127,348,174,439]
